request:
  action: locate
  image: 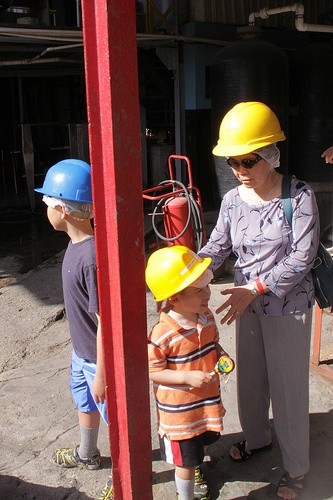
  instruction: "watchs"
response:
[248,284,259,299]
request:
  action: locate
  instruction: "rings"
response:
[236,311,240,315]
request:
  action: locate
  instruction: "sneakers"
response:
[50,443,101,470]
[91,476,114,500]
[192,468,211,500]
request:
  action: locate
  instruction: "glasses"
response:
[224,157,263,169]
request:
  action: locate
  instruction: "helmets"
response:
[211,101,286,157]
[33,159,93,205]
[145,245,212,302]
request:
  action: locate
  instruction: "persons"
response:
[32,158,110,471]
[144,246,231,500]
[196,101,333,500]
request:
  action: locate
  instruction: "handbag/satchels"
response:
[312,242,333,309]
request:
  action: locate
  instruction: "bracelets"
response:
[255,277,265,296]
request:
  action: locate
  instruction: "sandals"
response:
[229,439,274,462]
[275,471,309,500]
